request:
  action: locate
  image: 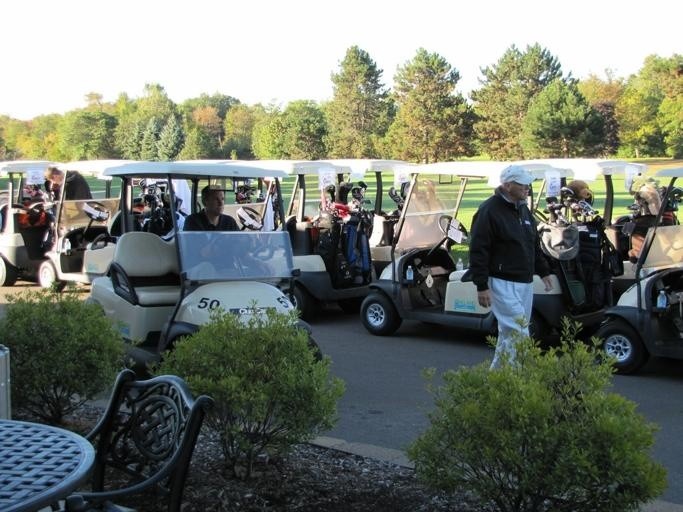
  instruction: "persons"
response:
[45,166,92,200]
[468,166,555,372]
[182,184,240,230]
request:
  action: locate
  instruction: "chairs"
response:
[56,367,216,512]
[108,209,182,306]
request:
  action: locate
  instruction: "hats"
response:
[638,186,661,216]
[500,164,537,186]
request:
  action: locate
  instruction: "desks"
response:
[0,419,98,512]
